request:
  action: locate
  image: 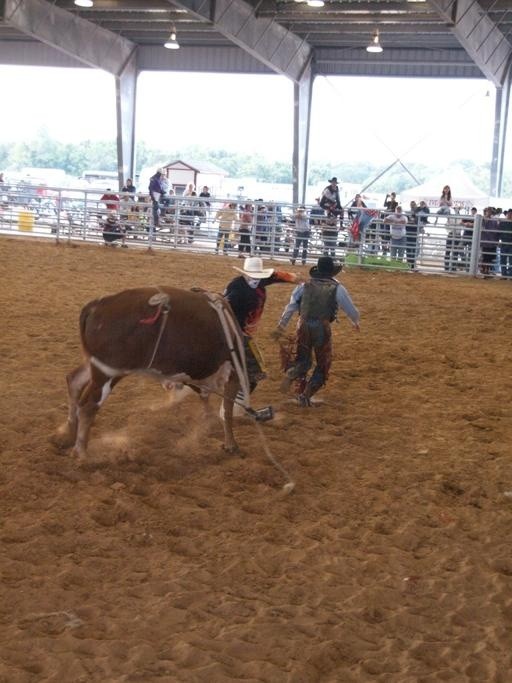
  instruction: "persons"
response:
[219,257,304,418]
[269,256,360,406]
[94,167,212,247]
[442,204,511,280]
[320,175,346,230]
[433,184,453,225]
[213,192,430,271]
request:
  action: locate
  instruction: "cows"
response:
[56,285,246,463]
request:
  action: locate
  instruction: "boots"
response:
[280,360,311,393]
[298,367,329,407]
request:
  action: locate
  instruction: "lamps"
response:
[366,24,384,53]
[163,12,180,49]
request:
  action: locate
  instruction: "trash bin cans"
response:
[18,211,33,232]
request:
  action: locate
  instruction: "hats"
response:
[157,168,167,175]
[309,256,343,278]
[483,207,512,214]
[328,178,339,184]
[232,256,274,279]
